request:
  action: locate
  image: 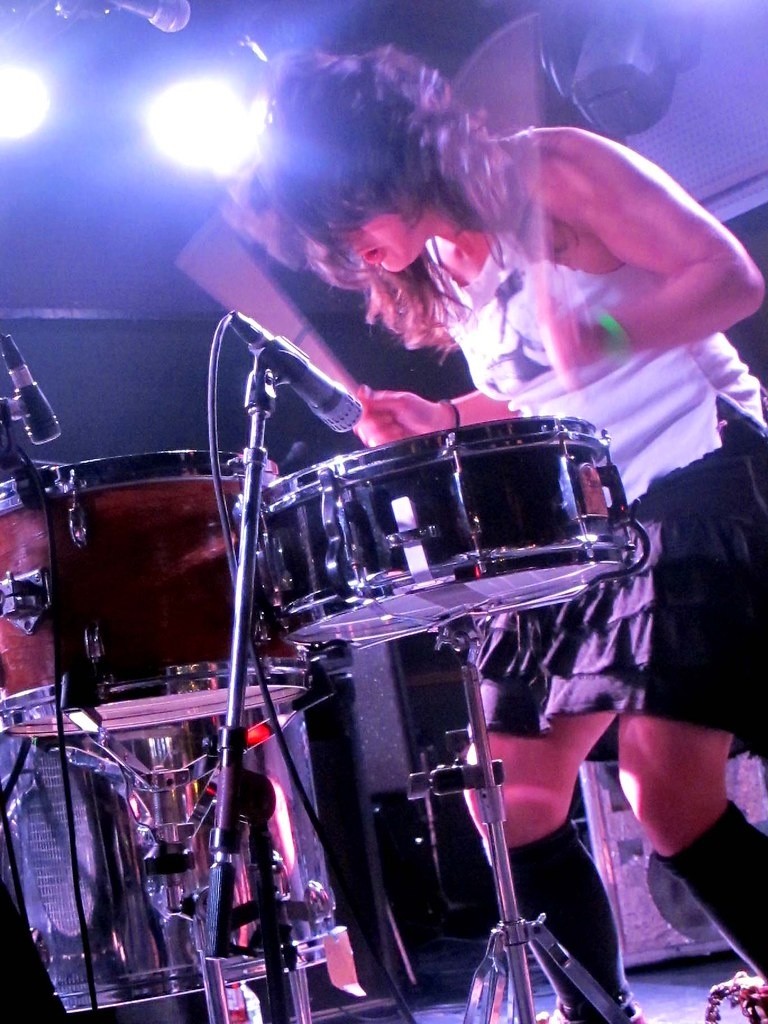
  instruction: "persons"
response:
[246,47,768,1024]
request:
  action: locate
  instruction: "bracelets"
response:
[443,399,467,433]
[598,312,630,355]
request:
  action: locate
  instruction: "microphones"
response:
[229,311,363,432]
[0,335,62,446]
[110,0,192,33]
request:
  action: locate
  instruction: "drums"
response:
[0,708,352,1015]
[229,413,636,645]
[1,441,312,738]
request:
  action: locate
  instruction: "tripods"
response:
[437,618,635,1024]
